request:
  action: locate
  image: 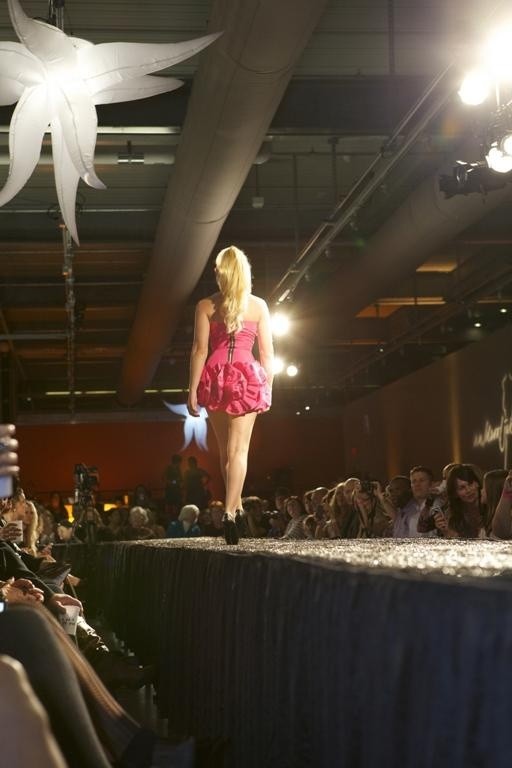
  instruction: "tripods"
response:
[66,497,107,543]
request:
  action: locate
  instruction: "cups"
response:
[7,520,23,543]
[58,604,81,636]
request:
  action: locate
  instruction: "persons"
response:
[241,460,512,542]
[185,245,274,545]
[0,421,222,767]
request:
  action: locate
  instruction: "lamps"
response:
[0,0,227,249]
[159,395,212,454]
[436,101,512,201]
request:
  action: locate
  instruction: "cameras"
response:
[359,482,375,494]
[431,506,440,514]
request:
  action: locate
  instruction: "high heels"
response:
[221,511,239,546]
[235,509,246,538]
